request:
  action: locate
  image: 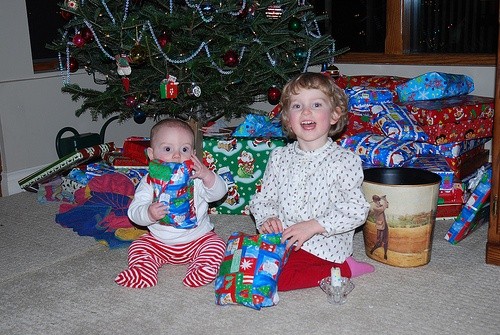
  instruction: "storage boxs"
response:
[18,71,495,243]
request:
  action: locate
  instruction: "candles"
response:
[331,260,341,287]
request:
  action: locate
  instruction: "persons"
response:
[114,118,228,289]
[249,71,376,291]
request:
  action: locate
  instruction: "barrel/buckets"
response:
[360,166,442,267]
